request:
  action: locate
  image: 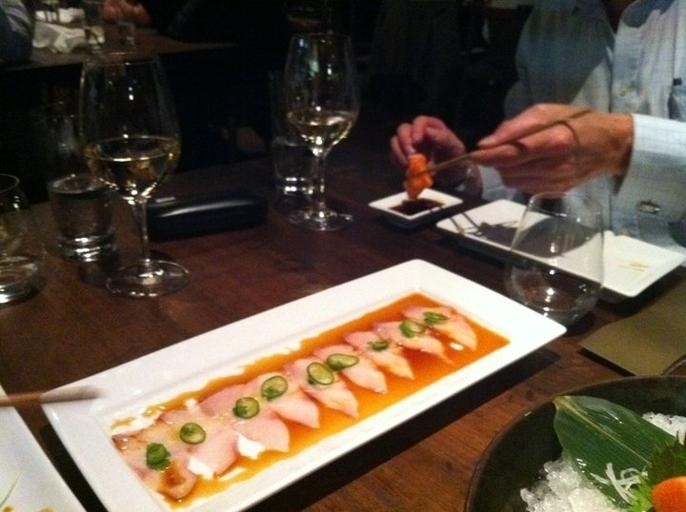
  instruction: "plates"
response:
[367,184,466,232]
[1,382,90,512]
[436,195,686,304]
[38,256,569,512]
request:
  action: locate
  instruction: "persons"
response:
[103,0,155,29]
[390,0,686,246]
[0,1,44,65]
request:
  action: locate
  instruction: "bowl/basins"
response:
[463,372,686,511]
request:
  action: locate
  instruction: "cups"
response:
[0,171,46,308]
[29,98,122,264]
[502,191,605,339]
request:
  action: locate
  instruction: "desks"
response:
[0,0,241,83]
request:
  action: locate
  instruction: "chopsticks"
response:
[404,104,593,181]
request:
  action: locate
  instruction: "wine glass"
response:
[277,30,364,236]
[76,50,192,302]
